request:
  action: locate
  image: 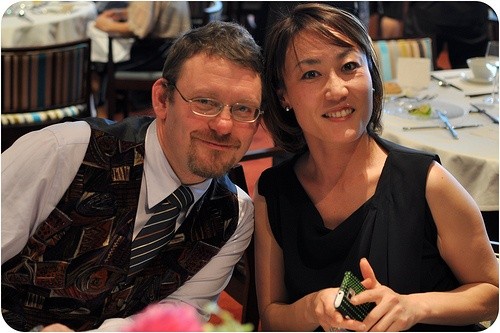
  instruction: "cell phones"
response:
[333,271,377,322]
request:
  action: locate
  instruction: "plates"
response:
[383,100,465,121]
[383,88,407,98]
[462,70,493,83]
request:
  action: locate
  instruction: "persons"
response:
[254,4,498,331]
[95,0,192,112]
[0,22,271,332]
[333,1,491,71]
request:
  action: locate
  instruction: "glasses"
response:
[160,76,264,123]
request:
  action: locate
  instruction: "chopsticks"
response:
[431,75,464,92]
[465,92,499,99]
[471,103,499,124]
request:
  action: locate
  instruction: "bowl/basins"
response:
[466,56,497,80]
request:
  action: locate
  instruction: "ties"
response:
[122,184,194,294]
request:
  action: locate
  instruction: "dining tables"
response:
[375,65,499,214]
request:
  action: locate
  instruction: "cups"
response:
[397,58,430,90]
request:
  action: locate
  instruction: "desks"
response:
[2,1,101,62]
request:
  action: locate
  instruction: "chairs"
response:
[374,36,435,83]
[1,38,93,154]
[104,1,224,120]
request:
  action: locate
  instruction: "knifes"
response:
[436,109,459,141]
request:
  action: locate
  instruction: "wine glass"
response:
[485,42,499,104]
